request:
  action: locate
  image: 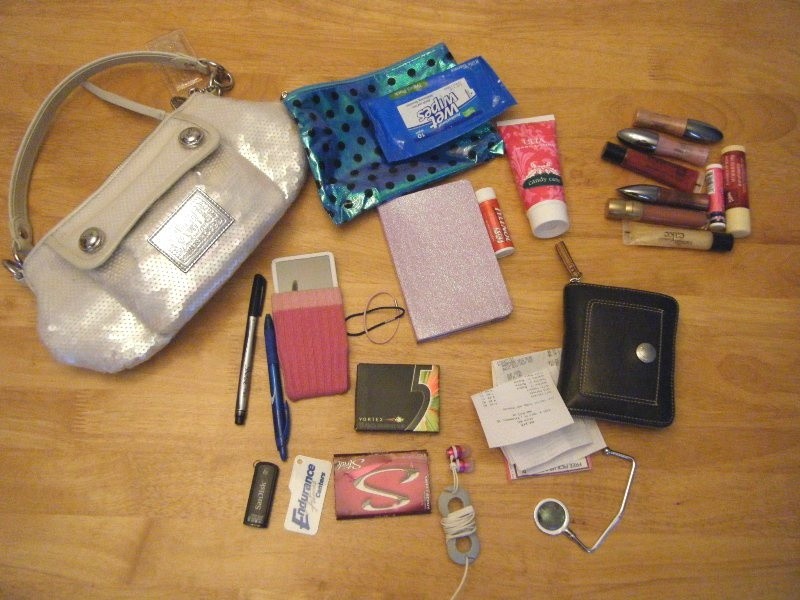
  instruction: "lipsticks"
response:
[475,187,515,259]
[601,111,751,253]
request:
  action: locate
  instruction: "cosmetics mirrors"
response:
[270,251,338,293]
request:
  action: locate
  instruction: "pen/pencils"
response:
[263,313,293,462]
[235,272,268,425]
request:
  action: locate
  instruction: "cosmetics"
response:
[497,114,569,238]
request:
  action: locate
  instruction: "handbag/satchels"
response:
[1,50,307,374]
[279,41,506,226]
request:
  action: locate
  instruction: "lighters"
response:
[242,462,280,528]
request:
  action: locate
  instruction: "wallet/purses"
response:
[354,363,441,432]
[559,278,678,428]
[332,449,431,520]
